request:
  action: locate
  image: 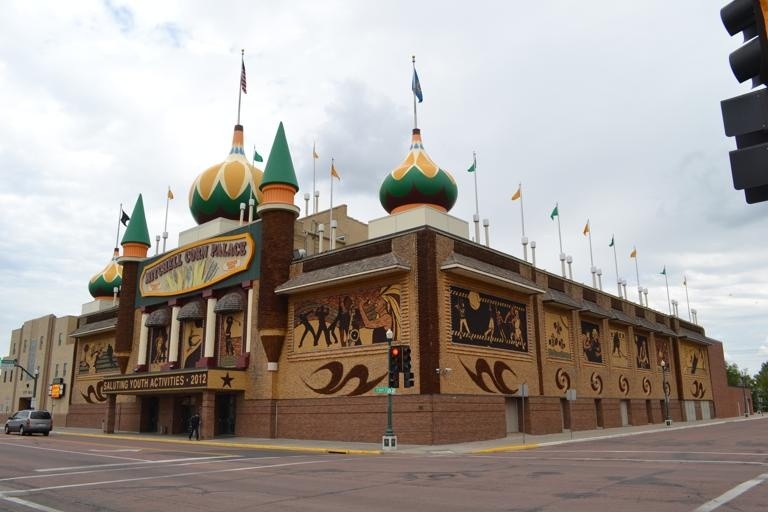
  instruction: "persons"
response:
[583,326,602,362]
[638,340,648,365]
[189,413,200,440]
[151,331,162,363]
[107,344,116,367]
[227,316,234,356]
[299,296,400,347]
[455,289,525,352]
[613,330,622,357]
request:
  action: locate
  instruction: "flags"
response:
[331,162,341,179]
[253,150,263,162]
[241,60,247,92]
[168,190,174,199]
[312,148,317,158]
[120,208,129,226]
[468,159,686,286]
[411,70,424,101]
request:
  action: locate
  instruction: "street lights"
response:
[8,364,38,410]
[382,329,397,450]
[741,374,749,417]
[660,360,672,427]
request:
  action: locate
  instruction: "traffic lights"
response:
[402,345,415,388]
[721,1,767,203]
[390,345,401,388]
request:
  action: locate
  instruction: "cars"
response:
[5,409,53,435]
